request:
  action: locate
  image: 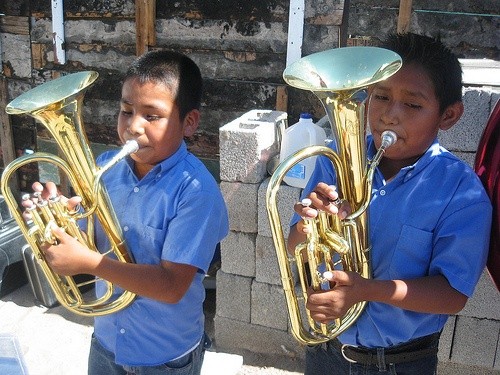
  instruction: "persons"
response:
[473,94,500,295]
[22,49,229,375]
[288,31,491,375]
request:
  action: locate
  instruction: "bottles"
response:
[279,113,327,189]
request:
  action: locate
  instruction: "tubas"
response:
[1,69,138,317]
[265,45,403,346]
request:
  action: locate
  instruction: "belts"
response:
[308,325,438,366]
